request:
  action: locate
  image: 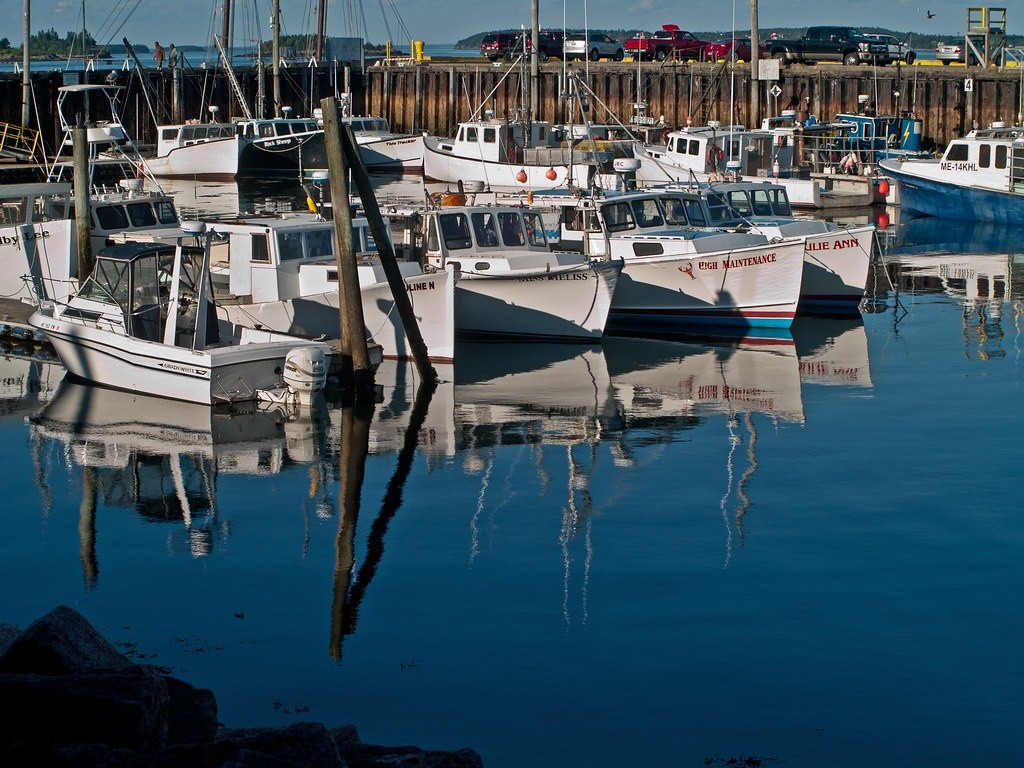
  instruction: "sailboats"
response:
[0,0,876,210]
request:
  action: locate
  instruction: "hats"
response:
[771,33,778,36]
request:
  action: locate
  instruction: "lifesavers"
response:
[888,134,898,146]
[707,146,723,165]
[508,150,520,163]
[663,129,673,143]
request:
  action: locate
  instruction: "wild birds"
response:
[925,9,936,19]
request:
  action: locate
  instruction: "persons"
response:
[152,41,166,70]
[168,43,178,69]
[771,33,782,40]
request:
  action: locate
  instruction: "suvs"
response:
[863,34,916,65]
[563,32,624,61]
[480,33,526,62]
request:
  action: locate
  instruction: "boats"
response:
[606,309,876,570]
[119,35,250,180]
[454,8,875,329]
[21,168,384,407]
[25,373,385,587]
[326,327,624,633]
[352,75,625,340]
[871,217,1024,361]
[0,85,182,309]
[878,57,1024,227]
[163,140,457,366]
[824,54,934,187]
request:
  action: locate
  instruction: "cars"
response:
[935,38,1001,66]
[705,39,765,62]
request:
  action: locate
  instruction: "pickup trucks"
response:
[625,24,712,62]
[522,30,569,63]
[762,25,889,66]
